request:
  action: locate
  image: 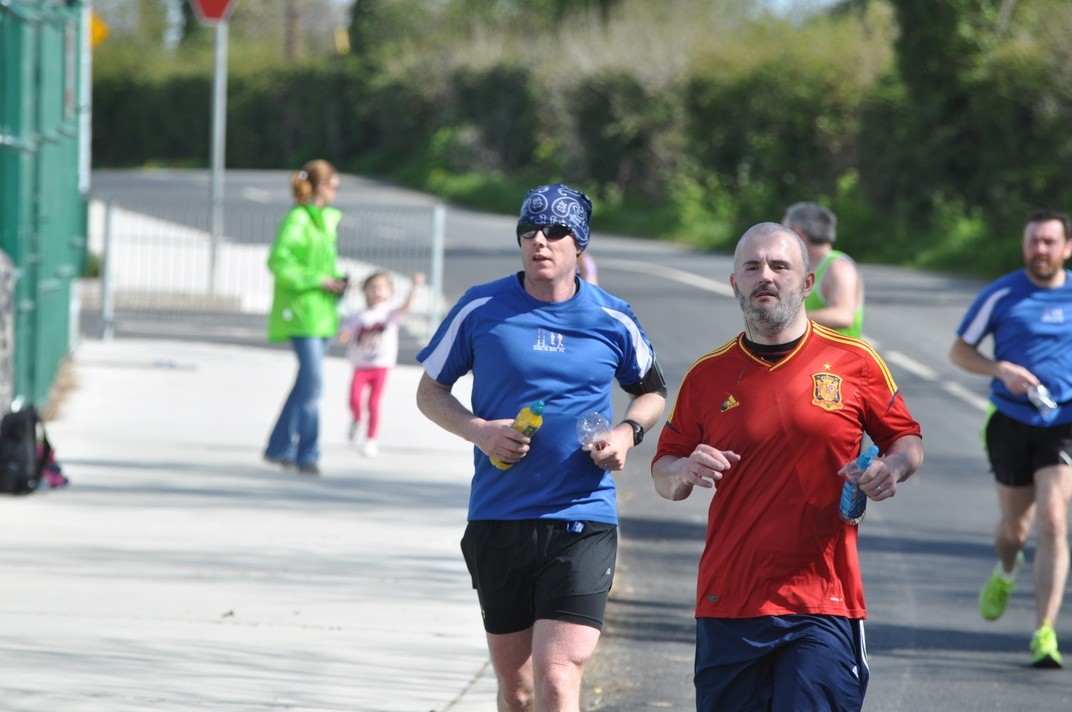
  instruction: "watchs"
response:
[622,418,644,446]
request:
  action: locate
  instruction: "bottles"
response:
[838,445,880,527]
[490,400,546,470]
[1026,382,1057,418]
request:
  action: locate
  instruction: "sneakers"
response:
[977,548,1024,618]
[1029,618,1063,667]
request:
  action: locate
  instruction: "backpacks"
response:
[0,405,51,494]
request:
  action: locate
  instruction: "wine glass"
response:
[577,413,617,475]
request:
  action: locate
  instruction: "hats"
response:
[516,184,592,255]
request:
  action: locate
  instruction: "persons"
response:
[946,209,1072,672]
[414,181,674,712]
[256,156,354,483]
[782,203,867,339]
[648,221,930,711]
[335,263,424,455]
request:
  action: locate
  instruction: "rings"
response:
[877,485,882,493]
[605,463,608,468]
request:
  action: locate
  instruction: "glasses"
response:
[517,221,572,240]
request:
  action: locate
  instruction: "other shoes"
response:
[295,464,321,477]
[348,424,361,441]
[361,438,378,457]
[264,452,292,471]
